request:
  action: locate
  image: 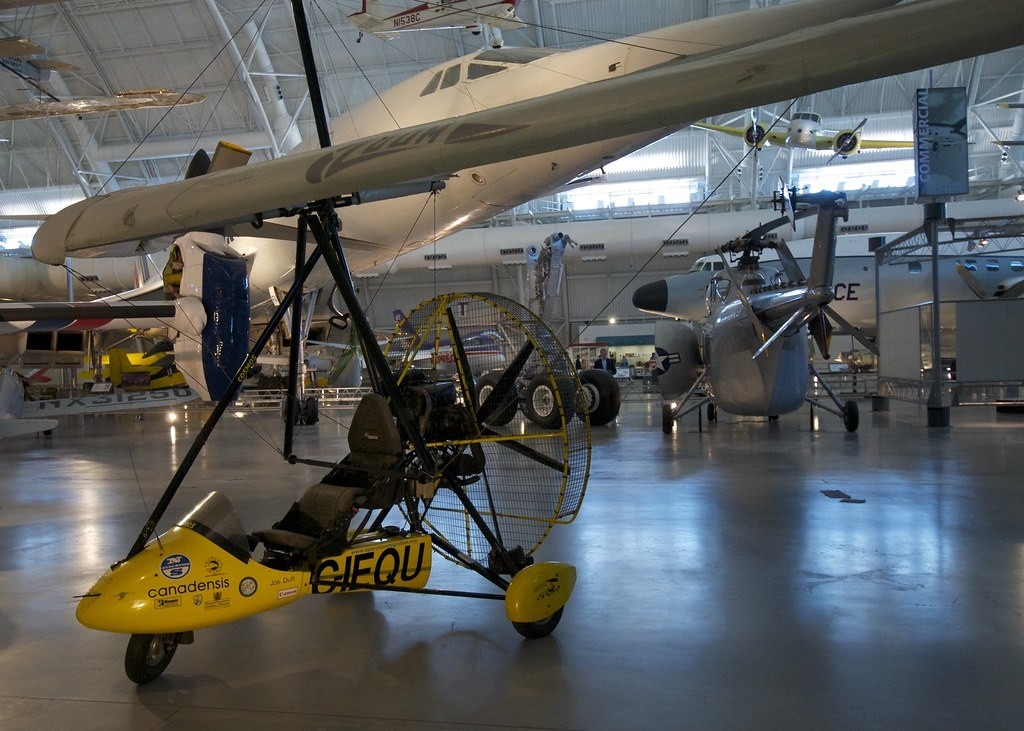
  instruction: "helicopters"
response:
[652,182,881,434]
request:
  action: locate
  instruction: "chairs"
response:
[263,394,398,550]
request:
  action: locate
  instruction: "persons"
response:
[280,322,286,338]
[621,356,628,366]
[650,353,655,360]
[576,355,582,369]
[610,355,616,363]
[594,348,617,374]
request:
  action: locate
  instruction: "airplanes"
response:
[0,0,1024,425]
[633,230,1024,336]
[690,108,915,165]
[381,309,506,378]
[346,0,528,41]
